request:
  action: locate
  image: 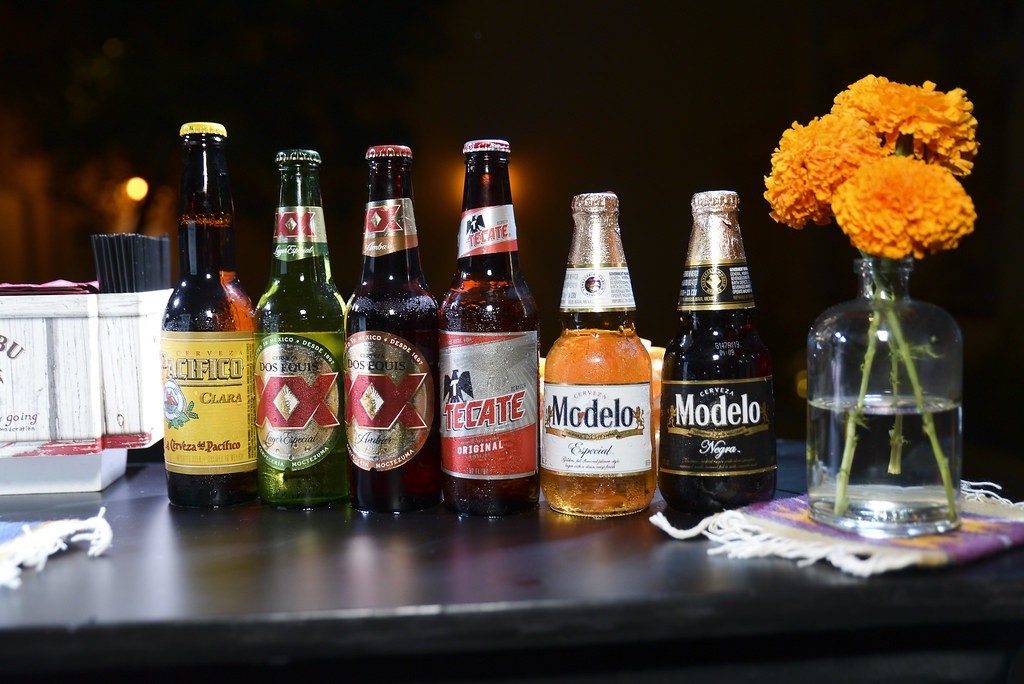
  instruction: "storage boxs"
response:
[0,287,175,457]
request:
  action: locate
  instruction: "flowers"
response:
[764,76,977,524]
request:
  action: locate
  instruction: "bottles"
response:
[540,193,657,516]
[658,191,778,514]
[161,123,261,509]
[254,149,350,510]
[343,146,440,514]
[440,139,540,516]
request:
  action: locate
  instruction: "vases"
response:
[807,255,961,541]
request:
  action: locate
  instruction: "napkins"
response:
[650,481,1024,578]
[0,506,113,588]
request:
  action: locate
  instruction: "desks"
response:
[0,440,1024,684]
